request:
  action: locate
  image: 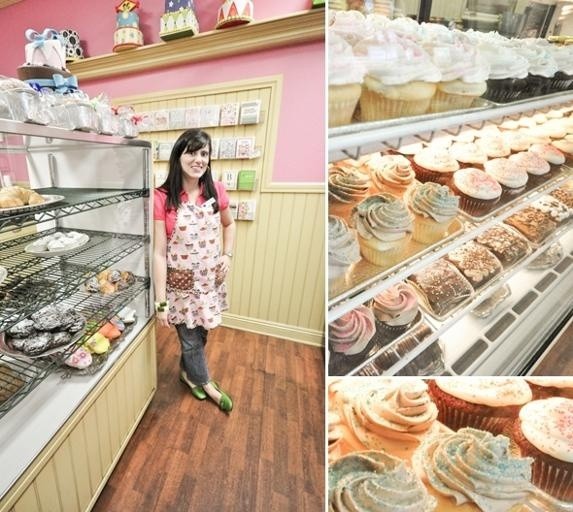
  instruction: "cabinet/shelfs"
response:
[327,88,571,378]
[0,117,165,511]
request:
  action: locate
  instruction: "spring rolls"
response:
[59,29,83,58]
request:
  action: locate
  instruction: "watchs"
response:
[223,252,234,257]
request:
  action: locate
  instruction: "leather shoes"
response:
[179,367,207,400]
[202,378,233,411]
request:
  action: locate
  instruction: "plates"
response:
[22,232,90,256]
[0,311,87,359]
[0,194,65,216]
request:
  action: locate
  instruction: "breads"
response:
[0,185,137,370]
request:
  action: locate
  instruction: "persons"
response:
[150,129,235,412]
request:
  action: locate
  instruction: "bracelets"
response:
[153,302,168,313]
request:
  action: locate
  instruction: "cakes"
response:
[160,0,200,31]
[328,8,573,512]
[217,0,253,22]
[113,12,144,44]
